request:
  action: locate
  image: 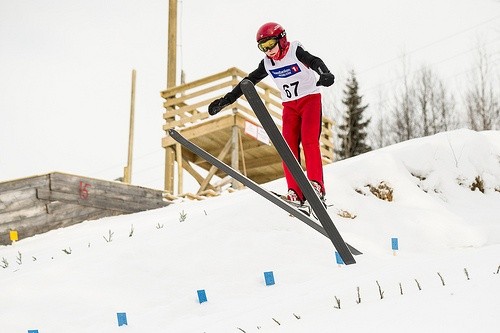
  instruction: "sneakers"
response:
[280,190,301,204]
[312,181,322,198]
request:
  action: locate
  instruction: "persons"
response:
[207,23,336,205]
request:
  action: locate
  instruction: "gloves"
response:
[316,73,335,87]
[208,97,231,115]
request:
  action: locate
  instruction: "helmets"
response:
[256,23,287,60]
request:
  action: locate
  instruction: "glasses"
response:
[258,36,279,52]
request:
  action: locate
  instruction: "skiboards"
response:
[167,79,363,265]
